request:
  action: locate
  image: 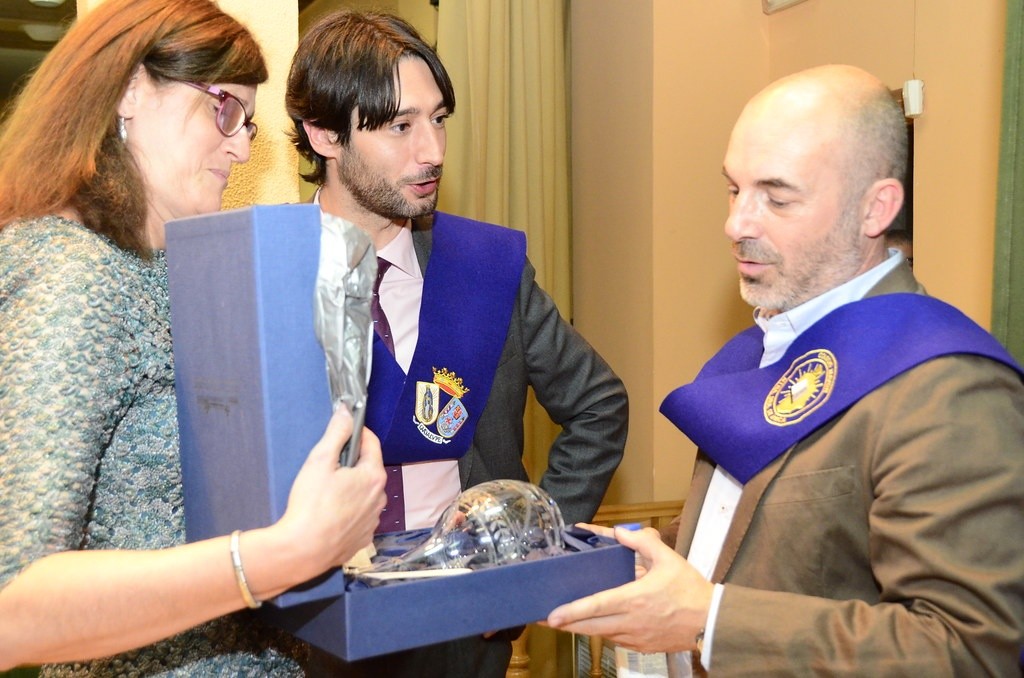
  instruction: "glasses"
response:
[185,81,258,142]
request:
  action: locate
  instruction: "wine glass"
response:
[347,478,567,585]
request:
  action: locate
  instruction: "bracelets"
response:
[231,531,261,608]
[695,629,705,653]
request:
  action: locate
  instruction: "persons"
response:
[285,12,630,678]
[0,1,390,678]
[546,64,1024,678]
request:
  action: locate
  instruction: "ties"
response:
[367,256,406,536]
